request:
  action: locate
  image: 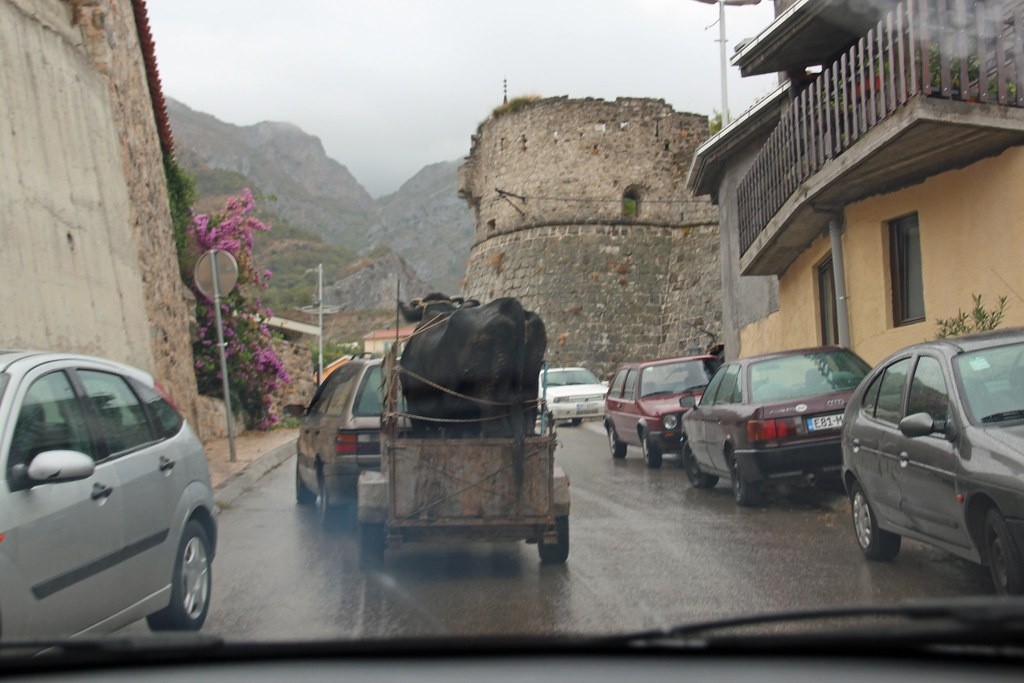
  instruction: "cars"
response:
[538,366,608,426]
[682,346,872,507]
[840,324,1023,597]
[295,355,410,526]
[0,349,220,657]
[605,355,723,467]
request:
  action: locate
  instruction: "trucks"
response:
[357,341,571,566]
